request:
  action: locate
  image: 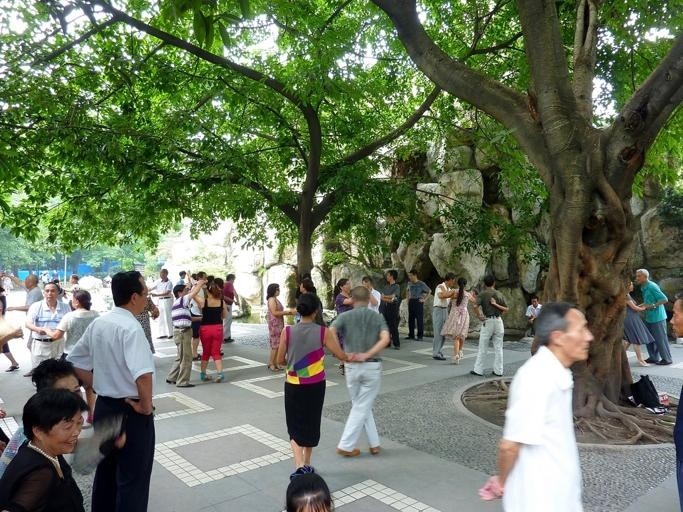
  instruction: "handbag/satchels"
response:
[629,375,665,409]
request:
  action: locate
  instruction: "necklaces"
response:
[27,441,60,467]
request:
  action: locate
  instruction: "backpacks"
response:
[629,374,670,409]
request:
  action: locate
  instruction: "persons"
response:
[166,269,240,387]
[433,272,456,360]
[329,271,401,457]
[66,271,156,512]
[0,386,87,512]
[147,268,173,340]
[524,295,543,336]
[405,269,432,342]
[634,269,673,366]
[283,464,334,512]
[0,359,126,482]
[266,274,348,465]
[470,275,509,378]
[440,279,478,365]
[0,272,101,424]
[621,282,654,368]
[670,290,683,512]
[135,299,159,353]
[478,300,594,512]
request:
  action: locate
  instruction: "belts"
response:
[435,306,447,309]
[345,358,382,363]
[35,338,57,341]
[226,303,233,305]
[174,325,192,329]
[485,315,497,319]
[159,297,171,299]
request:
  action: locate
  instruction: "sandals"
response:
[268,363,277,373]
[275,363,284,370]
[192,354,203,361]
[220,349,224,356]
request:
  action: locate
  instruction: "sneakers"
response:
[86,414,93,423]
[657,359,673,365]
[645,358,658,364]
[224,338,234,343]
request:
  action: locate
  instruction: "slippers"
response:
[6,365,20,372]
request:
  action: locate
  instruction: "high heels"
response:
[212,373,225,383]
[201,372,211,382]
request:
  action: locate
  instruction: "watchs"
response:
[653,303,656,309]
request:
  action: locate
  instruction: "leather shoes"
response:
[415,337,422,342]
[370,444,380,454]
[391,345,400,349]
[338,365,344,374]
[178,382,195,388]
[459,351,464,357]
[337,447,359,456]
[491,370,502,376]
[434,355,445,361]
[451,356,459,361]
[23,372,32,377]
[406,335,414,341]
[469,370,481,376]
[637,360,650,367]
[165,378,176,384]
